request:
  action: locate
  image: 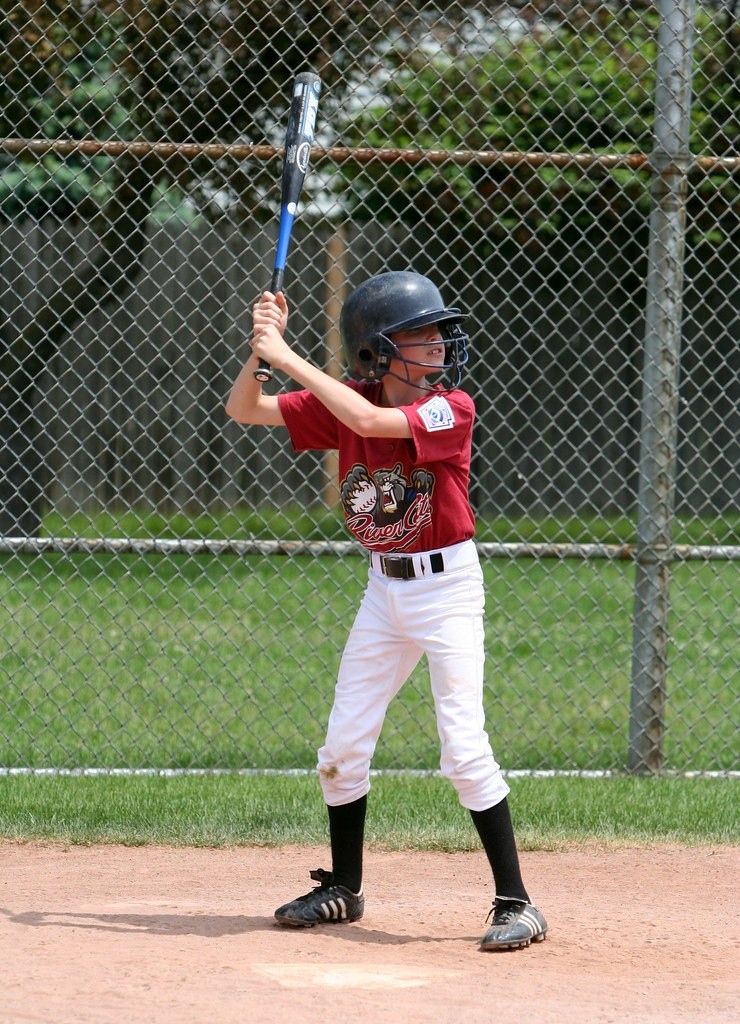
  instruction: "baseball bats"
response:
[252,71,323,382]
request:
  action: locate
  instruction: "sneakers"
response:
[480,896,548,949]
[274,868,364,927]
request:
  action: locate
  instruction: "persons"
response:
[225,271,549,952]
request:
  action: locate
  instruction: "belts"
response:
[370,550,444,580]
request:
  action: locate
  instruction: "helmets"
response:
[339,271,469,392]
